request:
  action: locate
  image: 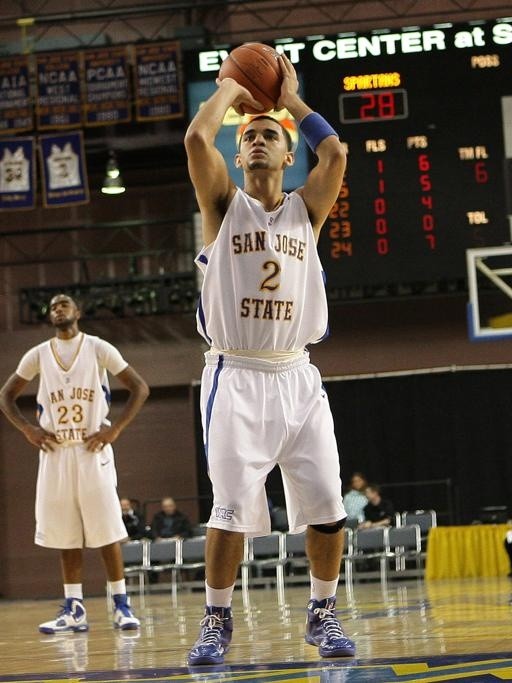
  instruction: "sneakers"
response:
[111,595,138,628]
[186,606,234,665]
[39,599,90,634]
[302,594,355,658]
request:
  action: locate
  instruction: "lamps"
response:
[100,155,126,196]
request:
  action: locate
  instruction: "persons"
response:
[184,52,356,664]
[120,498,148,542]
[0,293,149,635]
[358,484,396,571]
[148,496,191,583]
[342,472,369,522]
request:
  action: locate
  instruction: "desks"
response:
[426,523,511,580]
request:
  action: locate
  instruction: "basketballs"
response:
[218,42,284,114]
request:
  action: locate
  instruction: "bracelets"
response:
[297,111,340,153]
